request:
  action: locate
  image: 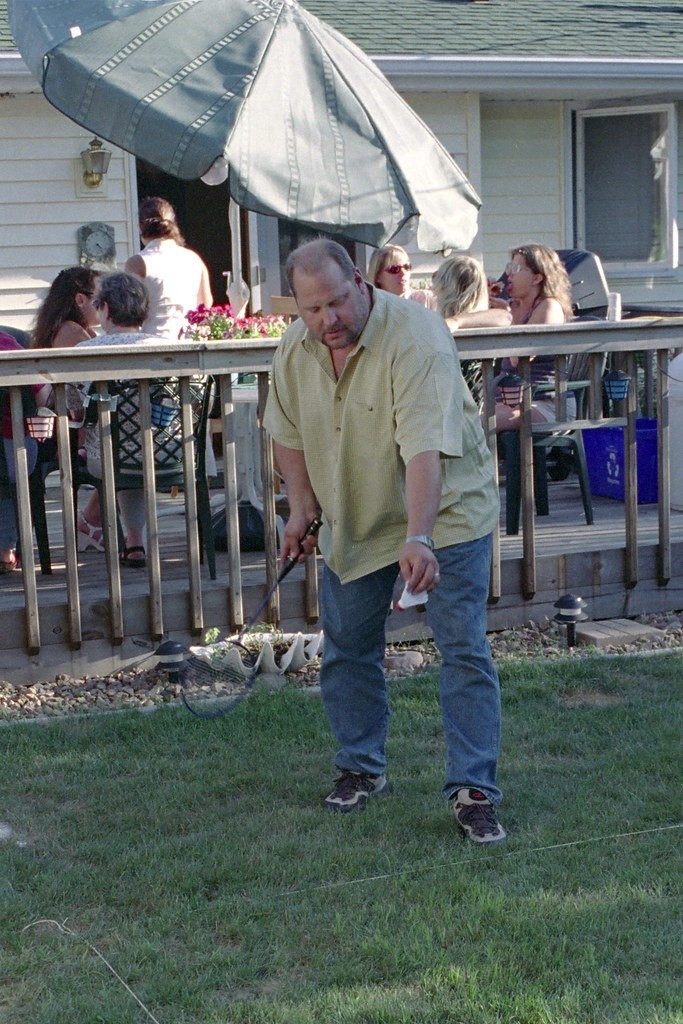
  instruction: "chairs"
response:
[70,373,218,582]
[0,388,60,576]
[500,314,610,533]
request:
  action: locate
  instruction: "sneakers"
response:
[323,772,387,812]
[453,789,509,845]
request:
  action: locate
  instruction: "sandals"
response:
[119,547,144,567]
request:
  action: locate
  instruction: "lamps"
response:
[553,593,590,647]
[153,640,191,684]
[74,135,112,199]
[604,368,632,401]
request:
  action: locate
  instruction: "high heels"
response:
[77,515,105,552]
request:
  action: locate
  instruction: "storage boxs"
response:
[582,418,658,506]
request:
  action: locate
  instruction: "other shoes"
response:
[0,555,17,573]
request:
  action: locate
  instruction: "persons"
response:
[0,269,204,574]
[364,242,577,473]
[124,197,216,489]
[258,238,508,843]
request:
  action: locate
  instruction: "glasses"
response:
[92,299,102,310]
[506,262,536,275]
[381,264,412,273]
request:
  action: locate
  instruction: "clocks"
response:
[77,220,117,270]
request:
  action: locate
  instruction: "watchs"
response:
[406,535,435,551]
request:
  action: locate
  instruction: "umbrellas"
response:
[6,0,481,319]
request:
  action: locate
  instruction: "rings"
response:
[434,573,440,576]
